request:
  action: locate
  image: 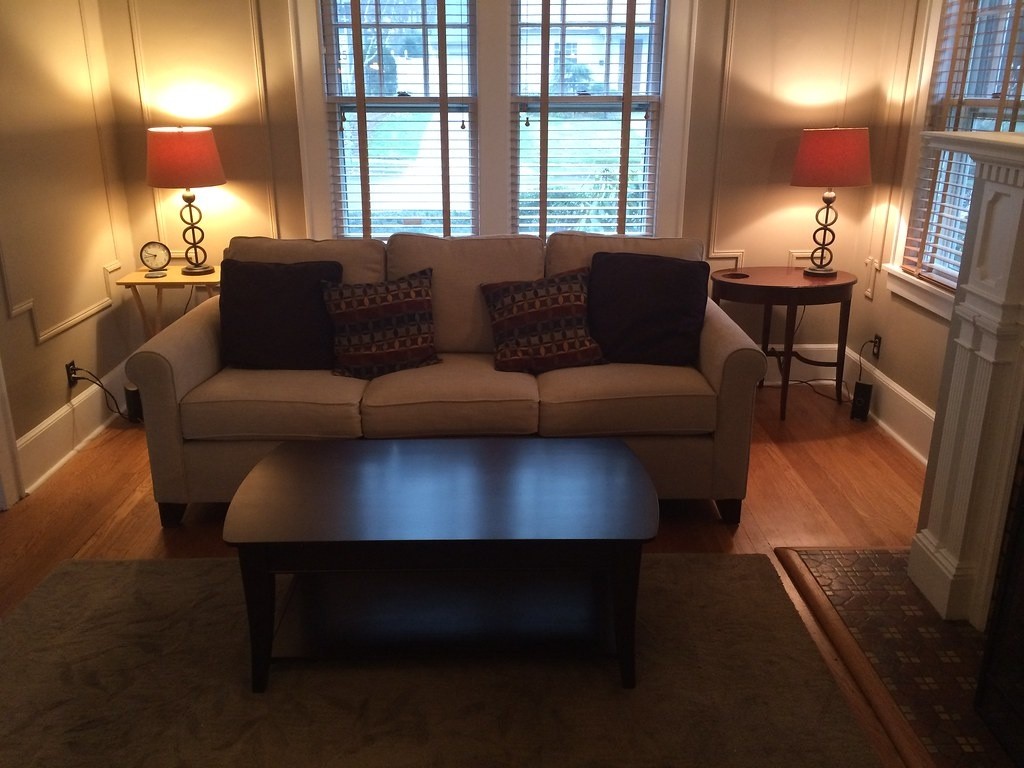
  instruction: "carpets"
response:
[0,553,882,768]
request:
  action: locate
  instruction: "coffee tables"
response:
[221,436,660,694]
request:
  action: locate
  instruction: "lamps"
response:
[789,125,872,278]
[146,124,227,276]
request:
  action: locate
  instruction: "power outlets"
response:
[66,360,78,389]
[872,333,882,360]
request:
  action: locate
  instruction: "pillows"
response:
[480,265,605,374]
[385,231,547,354]
[319,267,443,381]
[218,258,343,371]
[545,231,705,277]
[229,236,387,284]
[587,251,710,365]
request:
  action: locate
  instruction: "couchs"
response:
[124,249,770,531]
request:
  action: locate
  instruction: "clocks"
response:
[140,241,172,272]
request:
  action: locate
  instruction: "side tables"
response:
[711,266,857,421]
[116,265,221,342]
